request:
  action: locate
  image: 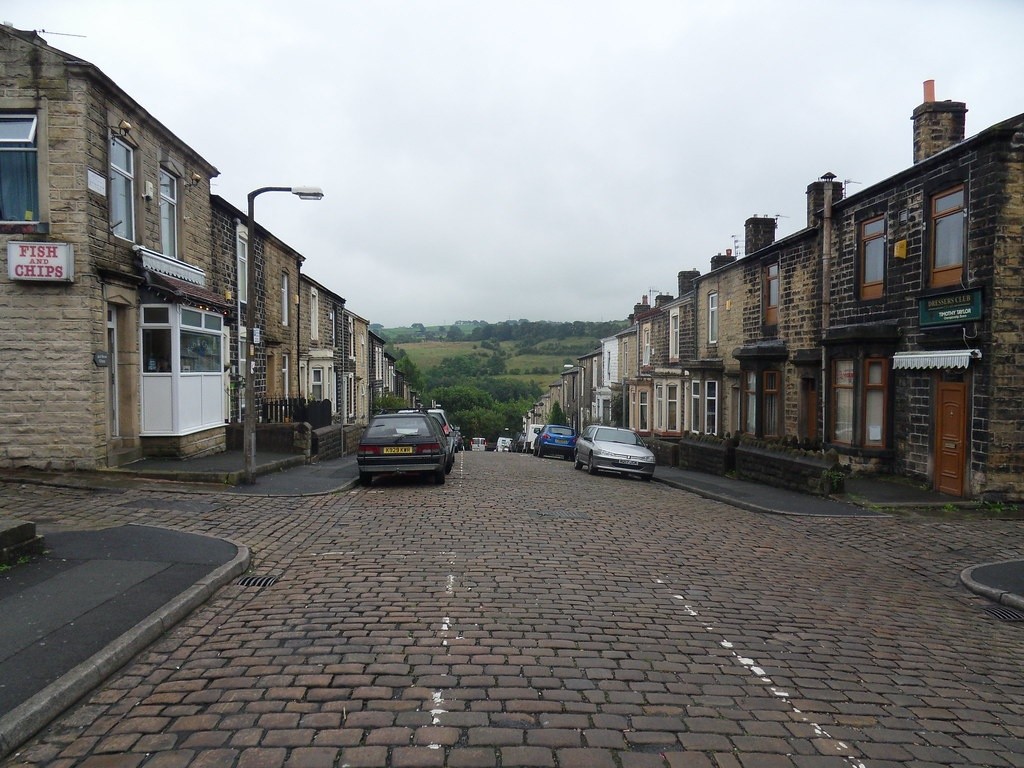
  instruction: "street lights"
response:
[242,184,325,486]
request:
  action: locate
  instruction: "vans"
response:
[524,424,545,454]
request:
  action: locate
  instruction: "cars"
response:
[573,422,656,481]
[398,410,527,456]
[356,407,453,488]
[532,424,575,461]
